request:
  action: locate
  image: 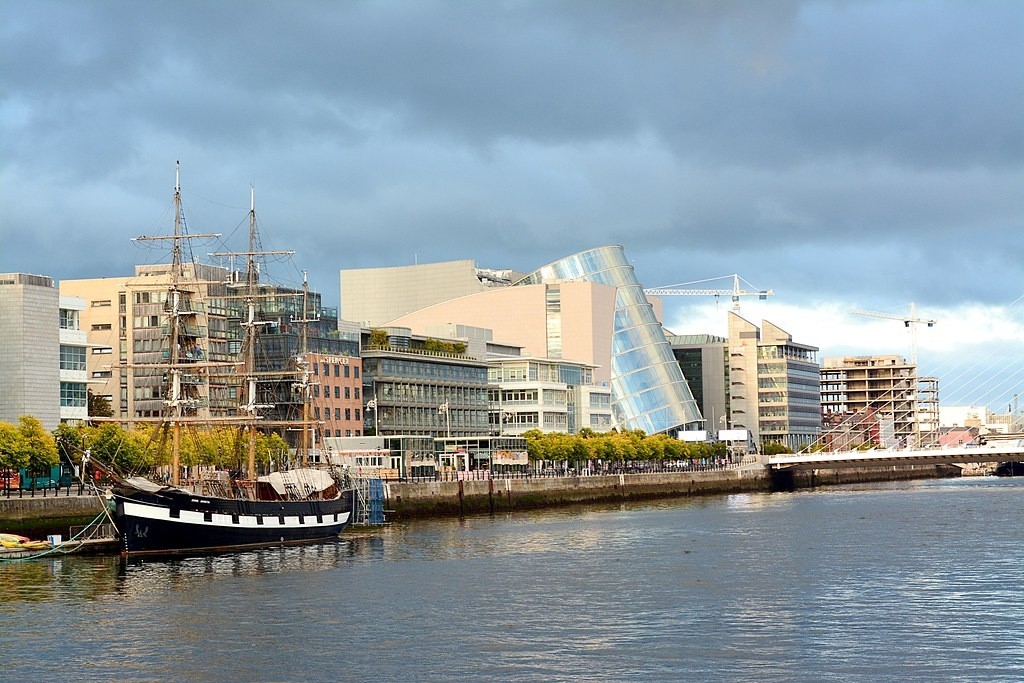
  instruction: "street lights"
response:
[719,416,728,461]
[367,400,379,469]
[81,435,88,494]
[437,403,450,439]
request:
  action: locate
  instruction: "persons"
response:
[546,453,729,479]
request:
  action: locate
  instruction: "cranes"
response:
[643,274,776,314]
[848,301,938,445]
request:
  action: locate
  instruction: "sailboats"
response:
[56,158,360,555]
